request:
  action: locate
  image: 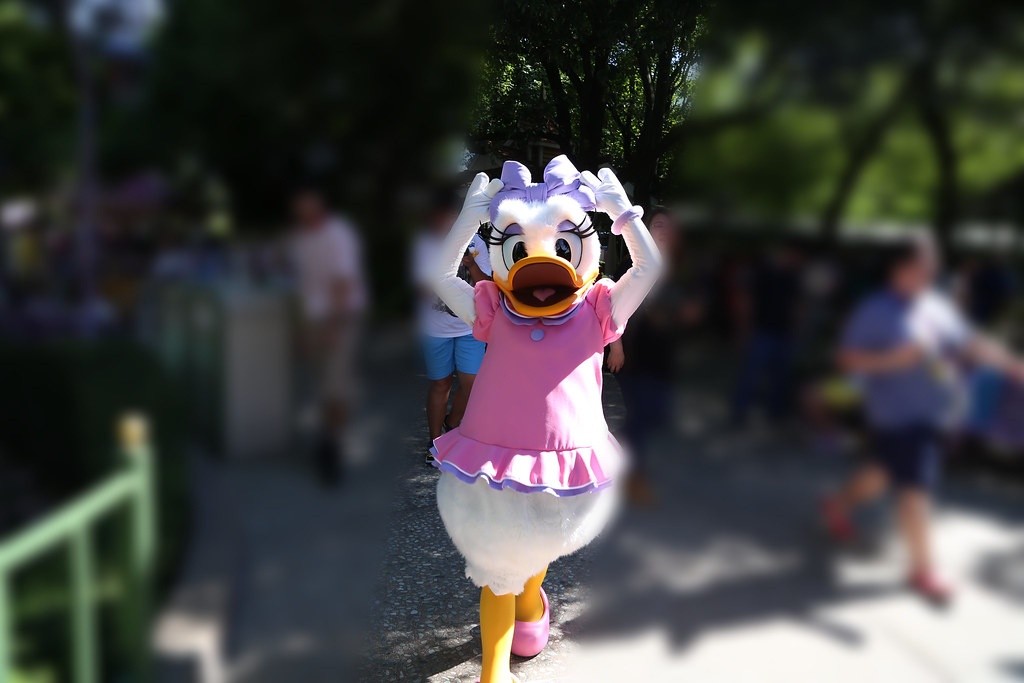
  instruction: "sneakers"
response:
[425,443,434,464]
[442,416,458,434]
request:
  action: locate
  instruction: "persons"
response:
[408,191,497,467]
[269,173,371,486]
[605,205,1023,611]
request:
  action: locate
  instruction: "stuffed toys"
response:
[428,151,667,683]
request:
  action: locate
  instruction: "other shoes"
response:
[909,575,948,612]
[512,586,550,658]
